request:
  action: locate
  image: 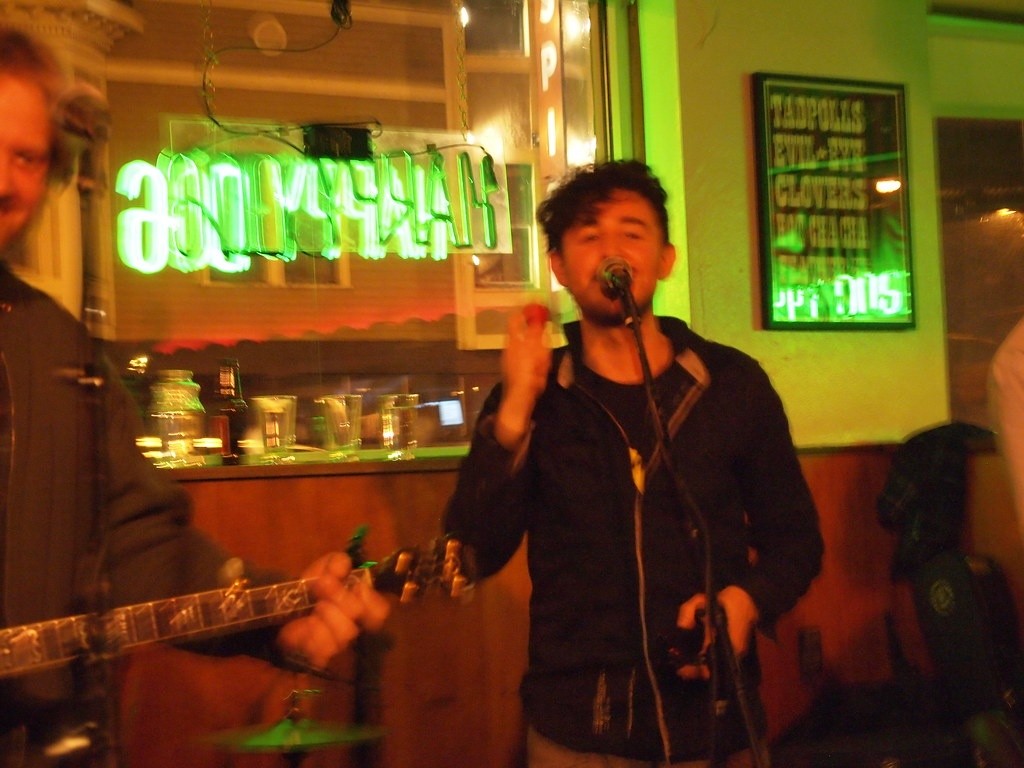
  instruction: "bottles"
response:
[145,367,208,467]
[213,358,247,465]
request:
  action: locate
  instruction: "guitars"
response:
[0,528,470,681]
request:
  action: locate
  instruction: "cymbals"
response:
[201,719,390,749]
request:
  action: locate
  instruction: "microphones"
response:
[596,257,633,290]
[52,83,112,142]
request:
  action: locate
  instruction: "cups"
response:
[254,395,297,463]
[323,395,362,461]
[379,393,419,461]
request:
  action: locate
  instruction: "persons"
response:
[438,160,826,768]
[0,31,395,768]
